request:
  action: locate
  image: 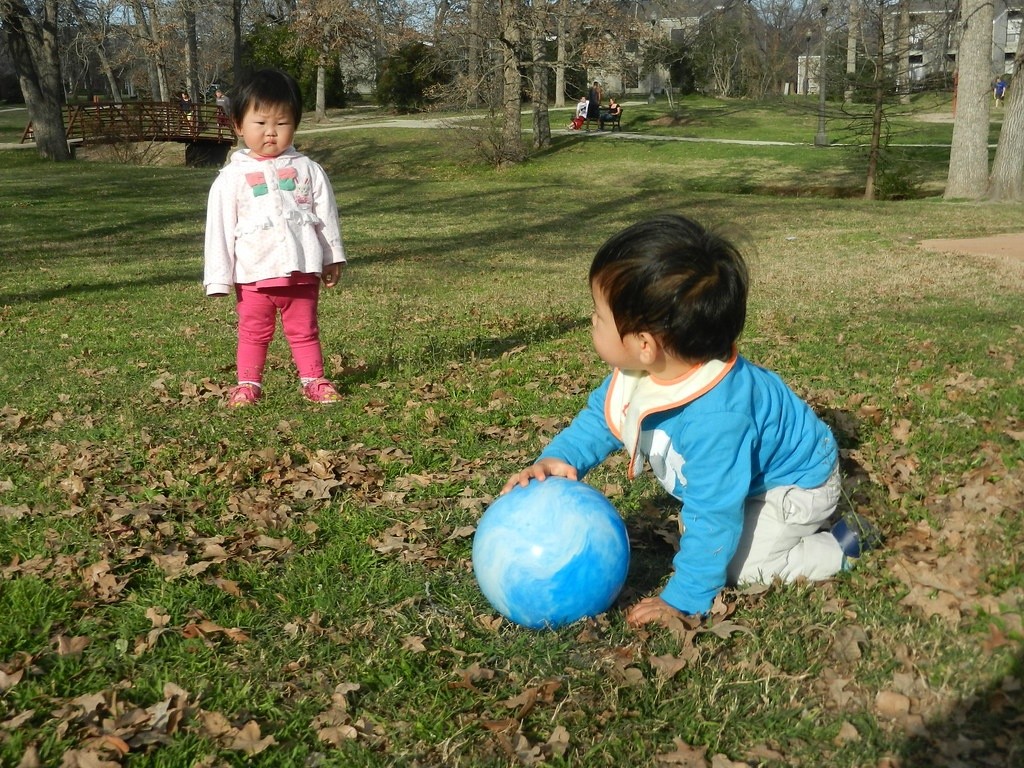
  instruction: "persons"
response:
[993,76,1007,108]
[596,96,621,133]
[213,90,236,145]
[178,92,194,136]
[567,97,591,131]
[586,82,601,133]
[500,217,886,627]
[202,64,346,404]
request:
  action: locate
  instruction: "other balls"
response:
[470,476,630,631]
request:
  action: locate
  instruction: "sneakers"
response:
[833,510,886,557]
[228,383,262,413]
[303,378,342,402]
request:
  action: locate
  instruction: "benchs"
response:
[570,106,624,133]
